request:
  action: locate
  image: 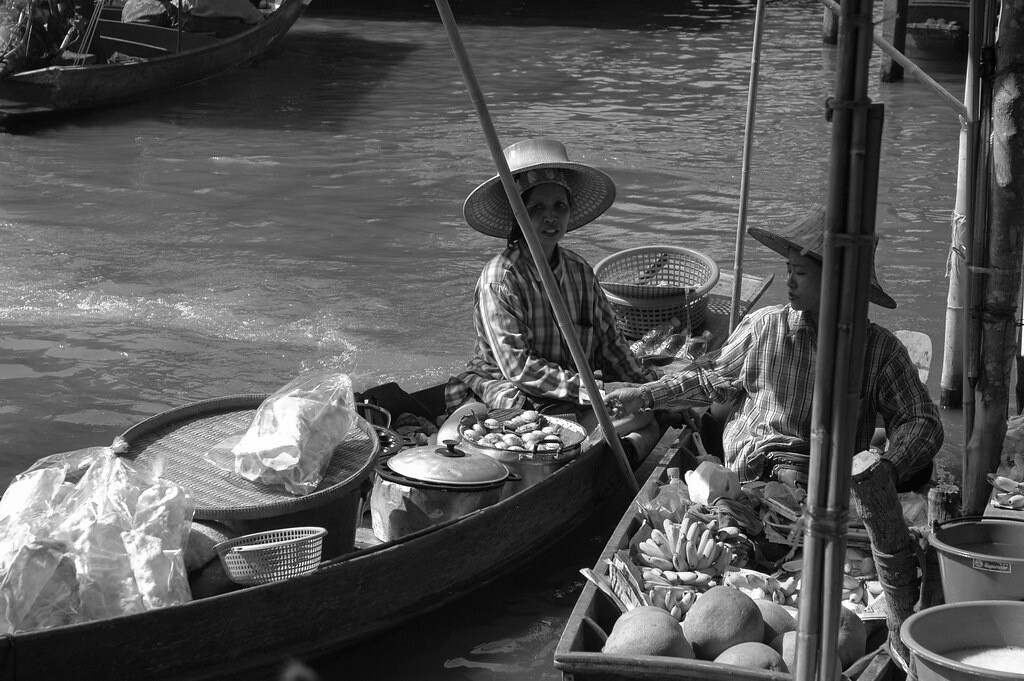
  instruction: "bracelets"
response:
[638,387,651,415]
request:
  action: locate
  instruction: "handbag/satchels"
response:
[762,450,810,495]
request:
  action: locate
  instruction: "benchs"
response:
[96,18,219,58]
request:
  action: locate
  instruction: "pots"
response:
[369,452,522,542]
[459,411,587,499]
[110,390,416,563]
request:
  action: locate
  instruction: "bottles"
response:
[675,329,713,360]
[630,318,681,358]
[655,328,693,357]
[663,468,683,487]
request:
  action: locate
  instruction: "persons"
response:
[605,203,944,490]
[446,139,703,433]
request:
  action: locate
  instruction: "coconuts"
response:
[600,585,866,673]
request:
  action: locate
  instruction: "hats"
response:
[747,202,897,309]
[463,139,616,240]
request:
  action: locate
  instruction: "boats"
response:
[549,327,932,680]
[900,0,973,66]
[0,255,776,680]
[13,0,305,116]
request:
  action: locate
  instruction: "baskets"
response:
[211,527,328,585]
[592,246,720,339]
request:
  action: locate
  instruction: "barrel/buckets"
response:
[928,517,1024,604]
[900,600,1024,681]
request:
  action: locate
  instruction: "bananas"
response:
[992,475,1024,508]
[637,512,885,621]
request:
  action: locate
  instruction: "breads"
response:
[464,410,561,451]
